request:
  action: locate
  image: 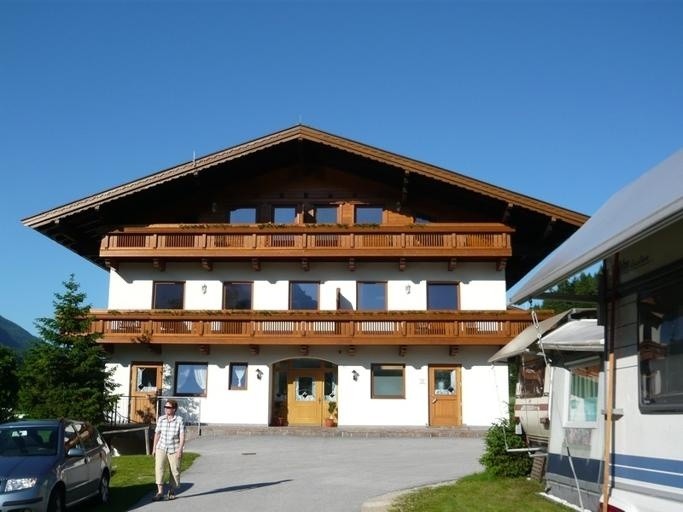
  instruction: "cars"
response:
[0,417,112,510]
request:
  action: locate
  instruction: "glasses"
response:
[164,406,173,409]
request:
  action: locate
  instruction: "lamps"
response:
[351,369,358,382]
[210,200,217,214]
[254,366,262,379]
[394,199,401,213]
[404,282,411,295]
[200,282,208,295]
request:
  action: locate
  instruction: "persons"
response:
[151,400,185,500]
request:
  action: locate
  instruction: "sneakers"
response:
[167,490,175,499]
[152,492,164,501]
